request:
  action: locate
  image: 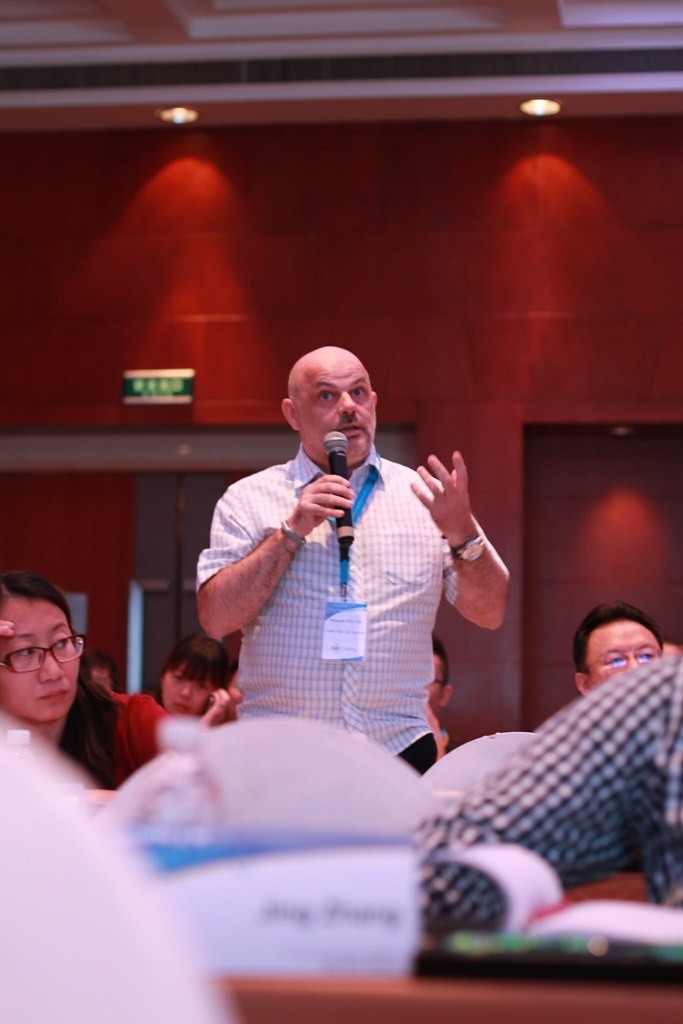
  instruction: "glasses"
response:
[0,623,86,674]
[583,646,661,677]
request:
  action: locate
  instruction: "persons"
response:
[196,346,510,776]
[0,572,683,949]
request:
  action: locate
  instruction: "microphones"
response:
[323,431,353,546]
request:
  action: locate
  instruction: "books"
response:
[438,842,683,948]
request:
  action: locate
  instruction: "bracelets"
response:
[282,521,306,545]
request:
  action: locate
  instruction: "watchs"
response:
[450,534,486,562]
